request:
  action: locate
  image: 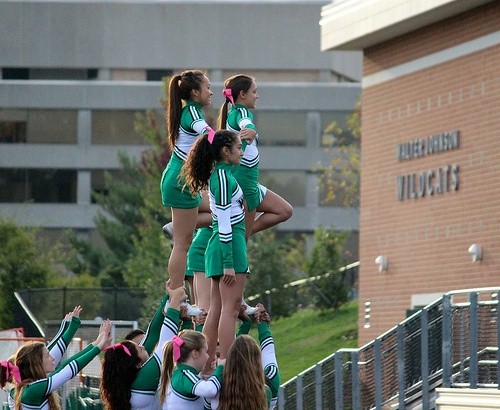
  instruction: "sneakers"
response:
[240,296,257,315]
[162,222,174,249]
[164,300,201,316]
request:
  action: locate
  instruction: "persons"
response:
[160,68,215,316]
[0,127,281,409]
[216,75,293,318]
